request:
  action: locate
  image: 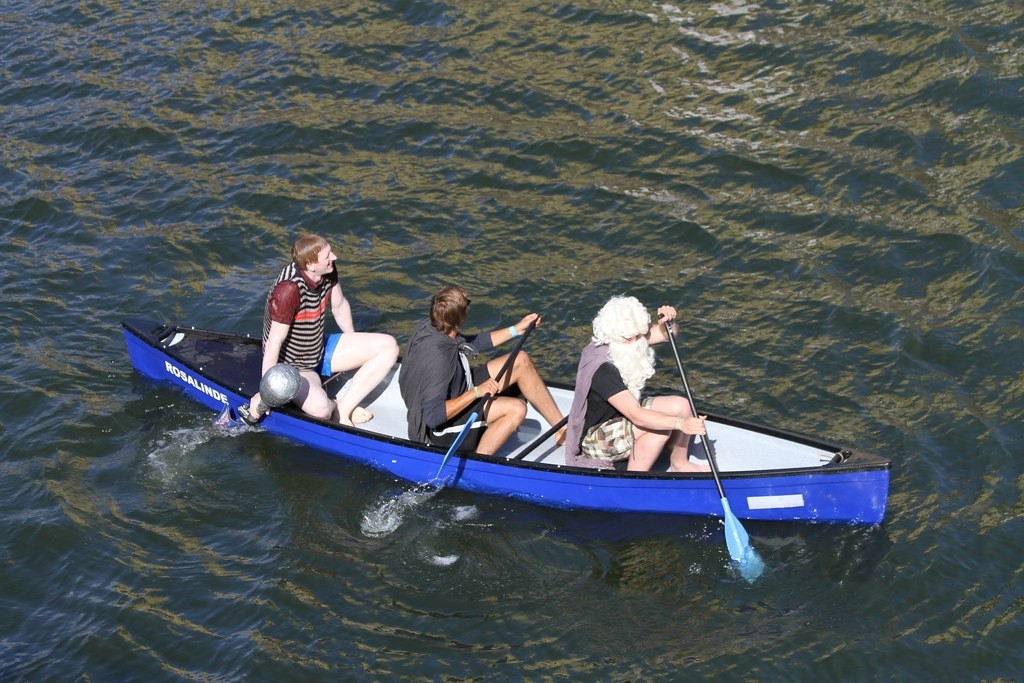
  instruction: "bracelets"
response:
[509,326,518,338]
[676,417,681,431]
[475,387,479,398]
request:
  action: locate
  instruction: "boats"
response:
[121,314,889,529]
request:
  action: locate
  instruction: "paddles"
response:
[436,321,535,483]
[658,312,749,559]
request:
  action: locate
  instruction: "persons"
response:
[398,285,566,454]
[566,296,711,472]
[250,234,400,427]
